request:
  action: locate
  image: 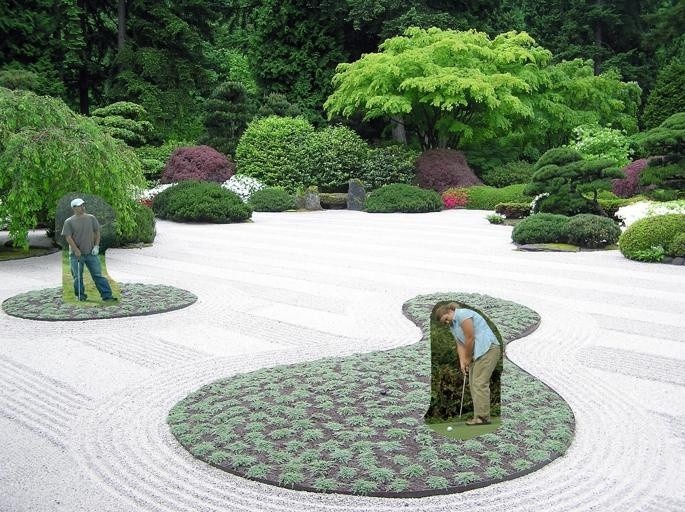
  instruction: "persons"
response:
[436,301,501,426]
[61,198,118,303]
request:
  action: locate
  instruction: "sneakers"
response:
[103,297,117,302]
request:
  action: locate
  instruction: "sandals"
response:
[465,415,491,425]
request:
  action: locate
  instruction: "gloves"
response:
[91,244,100,256]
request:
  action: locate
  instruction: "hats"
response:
[70,198,88,209]
